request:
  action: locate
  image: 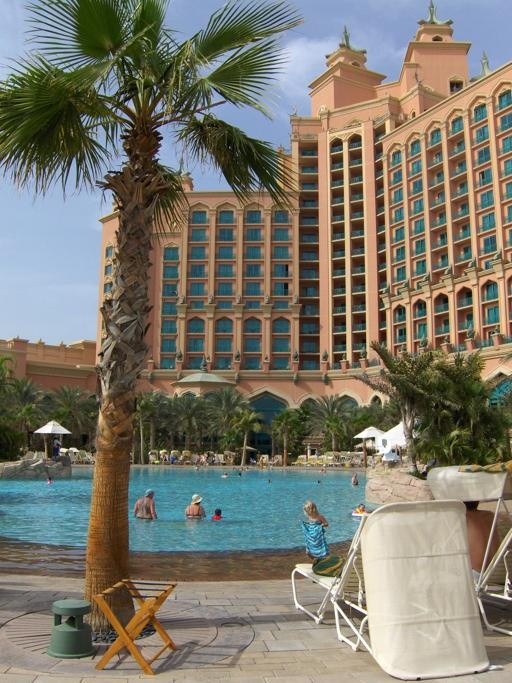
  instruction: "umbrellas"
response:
[34,419,71,457]
[382,422,417,446]
[354,426,385,447]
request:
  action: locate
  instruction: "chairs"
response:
[290,465,512,681]
[20,447,95,464]
[130,450,400,471]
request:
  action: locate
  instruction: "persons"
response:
[133,488,158,519]
[53,436,61,456]
[185,493,206,520]
[147,449,282,477]
[302,499,329,562]
[462,499,502,587]
[47,476,52,483]
[314,451,377,486]
[352,504,368,519]
[211,507,224,520]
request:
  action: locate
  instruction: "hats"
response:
[191,495,203,504]
[145,489,154,497]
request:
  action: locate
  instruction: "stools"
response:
[92,578,178,675]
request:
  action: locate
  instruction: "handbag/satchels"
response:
[312,553,345,577]
